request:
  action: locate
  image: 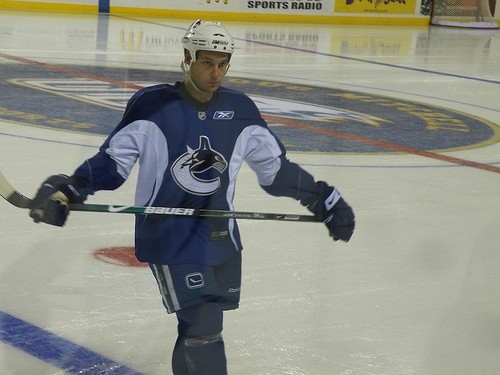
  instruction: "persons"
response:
[33,20,353,375]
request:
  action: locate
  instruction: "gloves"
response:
[29,174,85,226]
[308,180,355,242]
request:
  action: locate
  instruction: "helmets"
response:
[182,20,235,64]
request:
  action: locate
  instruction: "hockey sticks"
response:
[0,172,324,222]
